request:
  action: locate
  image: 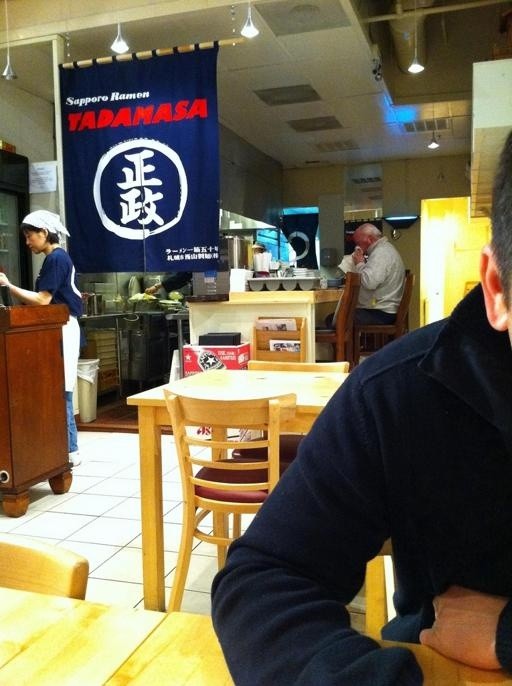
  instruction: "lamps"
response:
[239,0,259,39]
[0,0,17,81]
[109,0,130,55]
[407,0,425,74]
[427,98,441,148]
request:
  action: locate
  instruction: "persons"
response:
[294,343,300,351]
[262,323,273,330]
[289,343,293,351]
[251,241,267,255]
[273,343,280,350]
[144,270,192,295]
[325,222,406,356]
[275,323,287,330]
[0,208,84,467]
[280,343,287,351]
[209,118,511,684]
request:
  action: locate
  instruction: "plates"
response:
[159,303,182,308]
[294,266,341,287]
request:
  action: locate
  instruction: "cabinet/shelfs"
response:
[253,315,308,362]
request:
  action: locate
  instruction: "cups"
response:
[254,250,272,277]
[277,271,288,277]
[87,294,102,314]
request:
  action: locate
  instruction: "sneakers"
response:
[68,450,81,467]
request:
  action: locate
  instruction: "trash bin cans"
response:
[77,358,101,424]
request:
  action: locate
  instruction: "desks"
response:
[2,585,512,684]
[123,369,351,610]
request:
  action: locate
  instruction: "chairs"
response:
[162,386,294,612]
[313,273,362,361]
[1,536,89,601]
[354,268,416,366]
[248,359,349,373]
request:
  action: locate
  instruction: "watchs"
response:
[154,283,160,290]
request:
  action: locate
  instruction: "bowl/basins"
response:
[129,298,158,310]
